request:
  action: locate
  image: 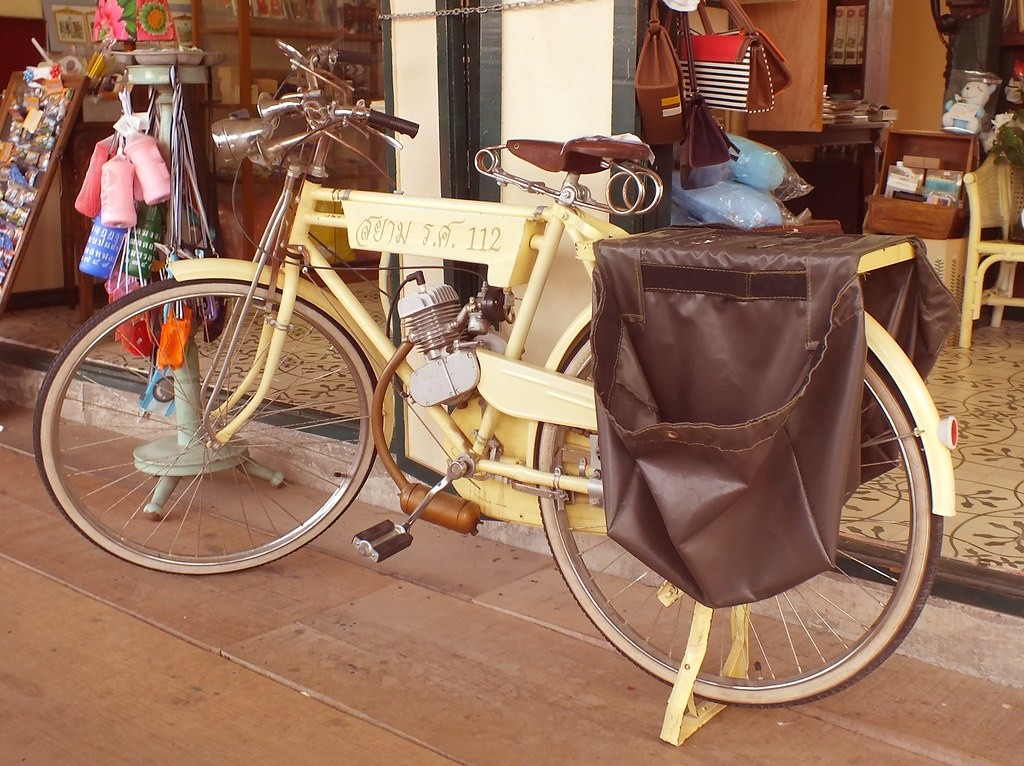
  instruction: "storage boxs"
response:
[926,240,967,350]
[866,127,980,240]
[869,108,897,121]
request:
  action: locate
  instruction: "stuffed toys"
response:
[942,81,997,133]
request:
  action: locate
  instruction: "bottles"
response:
[73,133,123,219]
[78,210,127,280]
[126,123,172,206]
[121,201,163,278]
[100,145,138,229]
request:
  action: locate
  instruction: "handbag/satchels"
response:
[634,0,686,145]
[677,0,793,113]
[680,11,740,190]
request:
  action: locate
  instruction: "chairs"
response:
[959,152,1024,349]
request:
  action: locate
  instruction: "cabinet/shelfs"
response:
[123,0,387,332]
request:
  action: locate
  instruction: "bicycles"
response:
[31,38,958,746]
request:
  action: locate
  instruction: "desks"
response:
[748,121,892,182]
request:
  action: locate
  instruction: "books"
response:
[823,102,868,124]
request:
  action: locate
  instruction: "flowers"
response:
[983,111,1023,168]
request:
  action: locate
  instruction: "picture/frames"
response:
[41,0,197,54]
